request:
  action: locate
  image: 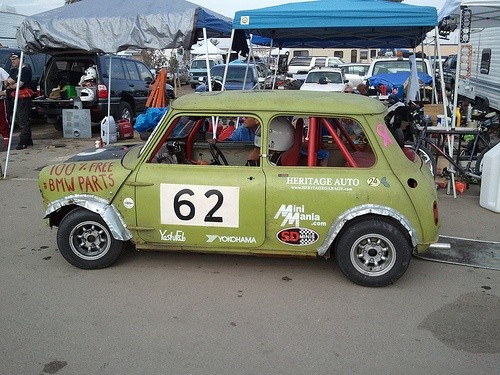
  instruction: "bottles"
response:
[456,107,461,127]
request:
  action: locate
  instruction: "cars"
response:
[368,56,433,84]
[36,92,441,289]
[299,66,349,93]
[337,63,384,86]
[205,63,265,90]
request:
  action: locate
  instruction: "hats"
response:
[9,51,25,60]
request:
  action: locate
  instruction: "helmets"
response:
[79,88,94,102]
[254,116,296,151]
[81,66,97,82]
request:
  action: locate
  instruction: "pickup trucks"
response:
[438,54,458,90]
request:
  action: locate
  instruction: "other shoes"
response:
[3,138,13,151]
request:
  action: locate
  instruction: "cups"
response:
[437,115,445,127]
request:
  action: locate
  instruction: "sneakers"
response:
[17,140,33,150]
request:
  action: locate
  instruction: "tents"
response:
[214,0,462,199]
[437,0,500,195]
[2,1,260,177]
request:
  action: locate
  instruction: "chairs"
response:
[280,117,305,166]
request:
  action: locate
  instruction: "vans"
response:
[187,55,224,90]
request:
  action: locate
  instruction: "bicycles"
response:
[403,101,500,186]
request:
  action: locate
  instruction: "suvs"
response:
[40,53,177,132]
[288,56,347,74]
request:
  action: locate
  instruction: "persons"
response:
[0,66,15,148]
[243,117,294,168]
[5,55,30,137]
[242,117,260,138]
[8,51,34,150]
[230,123,254,143]
